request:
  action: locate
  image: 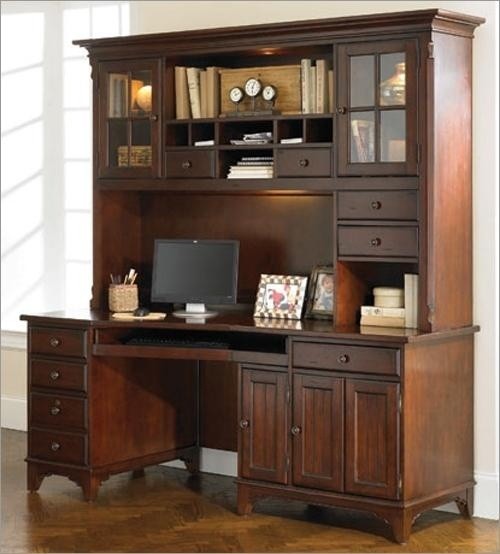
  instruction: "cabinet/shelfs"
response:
[69,7,488,335]
[26,323,201,501]
[237,339,481,546]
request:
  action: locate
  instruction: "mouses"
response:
[134,307,149,316]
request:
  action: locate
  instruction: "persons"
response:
[278,285,294,311]
[315,274,334,310]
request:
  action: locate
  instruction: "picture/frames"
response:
[254,317,304,332]
[303,263,333,323]
[251,272,309,322]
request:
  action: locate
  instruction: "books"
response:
[360,274,418,330]
[118,146,152,166]
[301,59,334,113]
[226,157,275,178]
[175,66,221,120]
[352,120,376,163]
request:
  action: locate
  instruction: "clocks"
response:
[261,85,277,101]
[230,86,245,104]
[245,77,262,98]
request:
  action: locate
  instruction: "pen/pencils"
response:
[108,267,139,285]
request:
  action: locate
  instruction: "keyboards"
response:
[125,335,235,349]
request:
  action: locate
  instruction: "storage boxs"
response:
[219,64,304,118]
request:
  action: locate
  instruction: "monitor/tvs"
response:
[150,239,240,317]
